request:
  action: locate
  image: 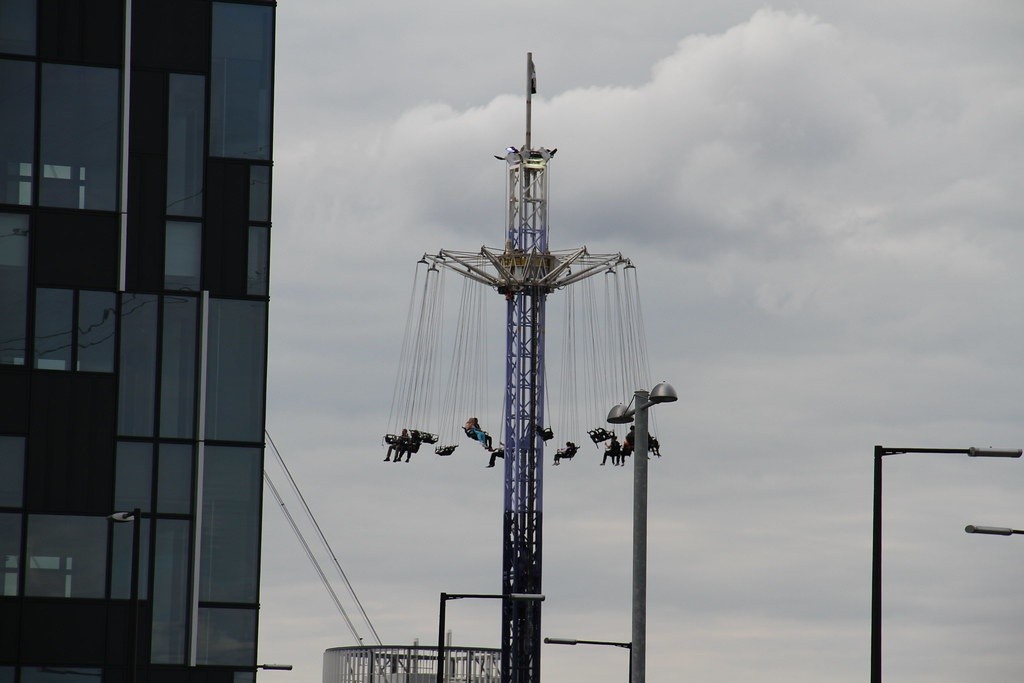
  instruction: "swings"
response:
[385,250,659,458]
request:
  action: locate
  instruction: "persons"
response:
[385,409,659,474]
